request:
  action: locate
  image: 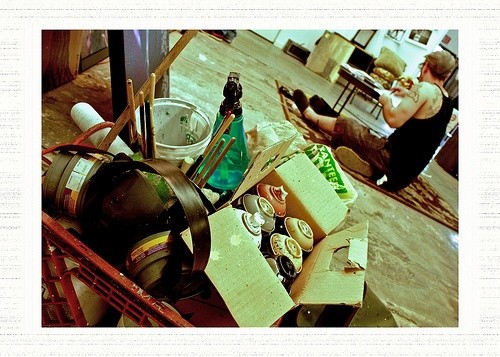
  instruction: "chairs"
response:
[349,57,394,120]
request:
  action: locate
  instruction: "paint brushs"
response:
[125,72,156,160]
[185,110,237,190]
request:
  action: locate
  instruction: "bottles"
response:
[234,183,314,293]
[195,72,252,208]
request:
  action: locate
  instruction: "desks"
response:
[333,65,384,121]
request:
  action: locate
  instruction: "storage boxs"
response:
[184,132,369,328]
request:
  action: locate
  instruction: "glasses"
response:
[419,63,426,69]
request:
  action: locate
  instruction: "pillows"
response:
[370,45,407,91]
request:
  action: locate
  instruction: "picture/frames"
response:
[384,29,435,50]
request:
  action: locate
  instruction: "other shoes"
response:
[336,146,373,177]
[294,89,313,121]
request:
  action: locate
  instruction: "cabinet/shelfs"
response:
[283,39,310,65]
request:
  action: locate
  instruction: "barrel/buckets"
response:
[129,98,214,173]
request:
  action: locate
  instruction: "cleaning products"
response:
[195,72,250,208]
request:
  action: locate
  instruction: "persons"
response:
[293,50,455,193]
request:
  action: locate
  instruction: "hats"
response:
[425,50,456,77]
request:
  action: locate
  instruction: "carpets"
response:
[275,78,458,233]
